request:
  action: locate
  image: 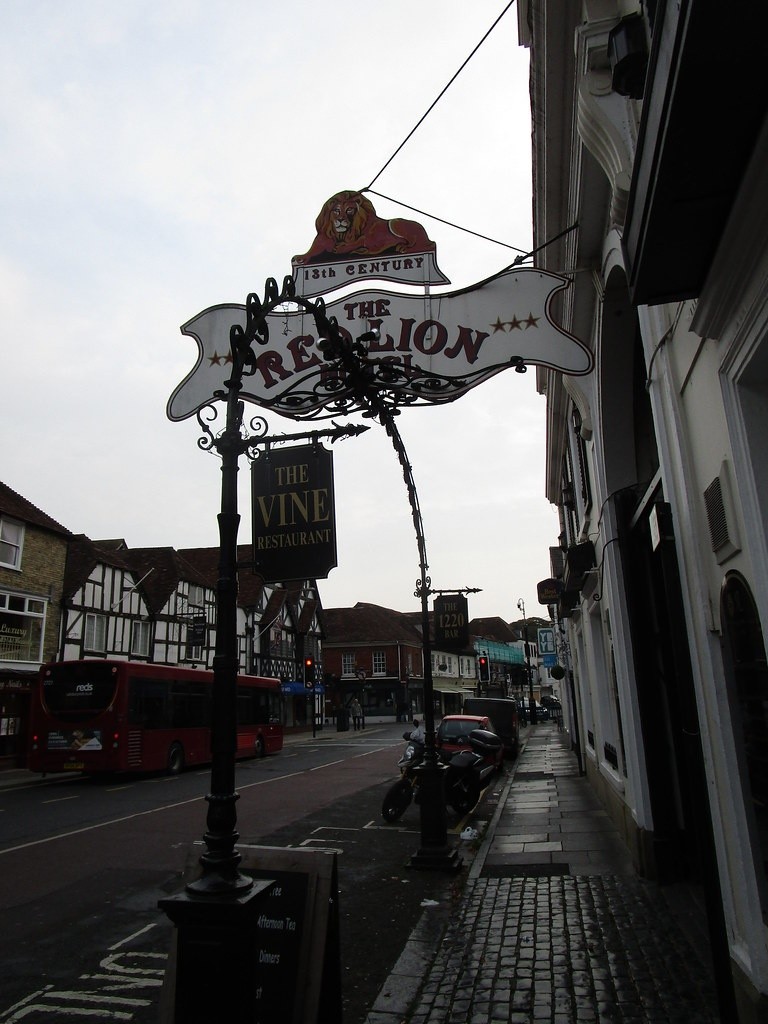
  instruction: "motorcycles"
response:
[381,719,503,824]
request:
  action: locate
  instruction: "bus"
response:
[33,658,283,780]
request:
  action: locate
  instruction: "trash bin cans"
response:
[336,707,351,732]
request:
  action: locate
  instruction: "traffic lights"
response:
[479,655,491,682]
[305,653,316,738]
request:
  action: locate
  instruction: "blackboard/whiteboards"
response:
[234,868,317,1024]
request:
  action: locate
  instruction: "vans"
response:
[462,697,520,759]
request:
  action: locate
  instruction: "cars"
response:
[434,714,495,755]
[539,695,562,711]
[517,696,550,724]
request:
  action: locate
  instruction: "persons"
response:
[351,697,364,731]
